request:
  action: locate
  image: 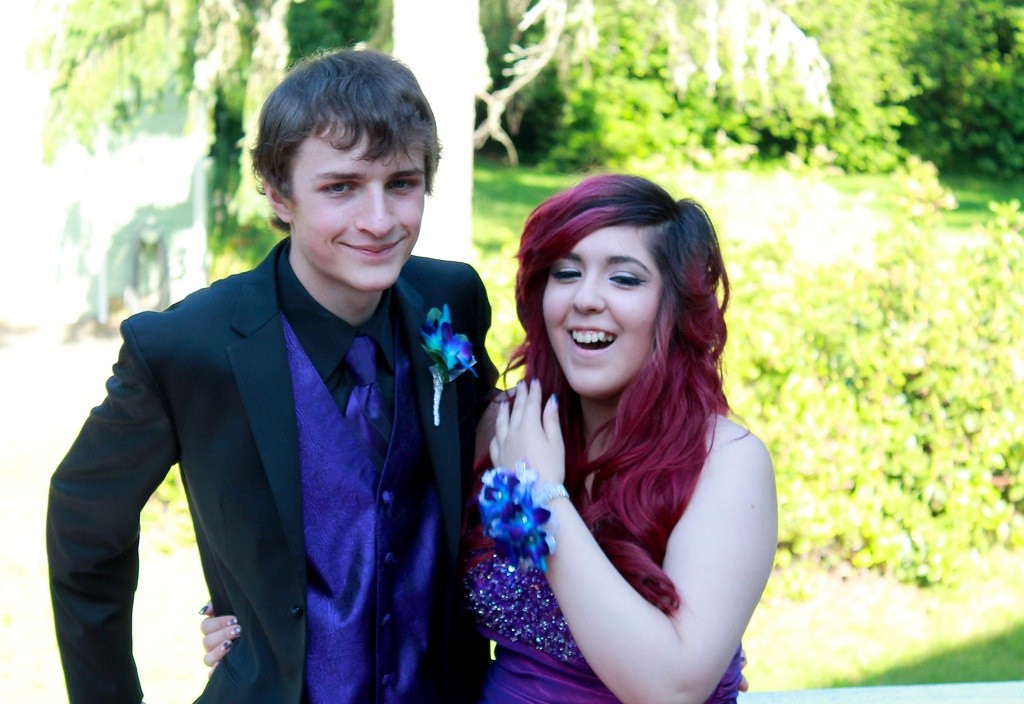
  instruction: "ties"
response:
[345,336,388,461]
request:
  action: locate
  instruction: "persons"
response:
[199,175,779,704]
[45,50,749,704]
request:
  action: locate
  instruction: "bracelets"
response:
[532,483,571,507]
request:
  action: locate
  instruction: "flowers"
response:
[419,303,478,427]
[479,462,557,574]
